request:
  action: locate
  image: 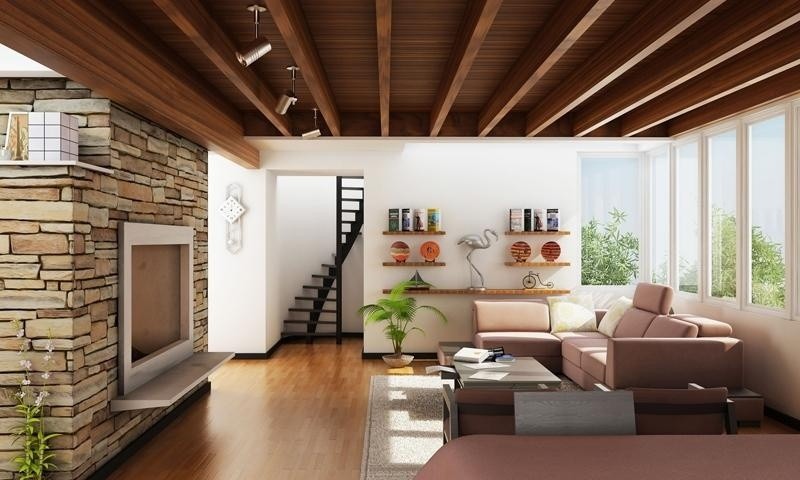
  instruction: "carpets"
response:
[359,372,586,479]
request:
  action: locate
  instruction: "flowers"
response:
[0,318,58,479]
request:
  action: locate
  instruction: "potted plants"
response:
[358,280,448,368]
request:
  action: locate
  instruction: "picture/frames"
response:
[0,111,28,160]
[5,112,29,160]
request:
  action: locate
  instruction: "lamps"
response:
[233,3,272,68]
[302,108,322,140]
[274,65,301,115]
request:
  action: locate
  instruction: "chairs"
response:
[514,390,637,436]
[593,382,739,435]
[441,383,561,445]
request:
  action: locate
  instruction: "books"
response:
[453,345,514,365]
[509,208,559,232]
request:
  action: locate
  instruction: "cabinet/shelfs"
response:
[382,230,571,295]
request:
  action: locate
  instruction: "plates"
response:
[511,242,531,262]
[420,240,440,262]
[391,241,411,263]
[541,242,561,261]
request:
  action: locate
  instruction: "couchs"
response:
[472,281,765,429]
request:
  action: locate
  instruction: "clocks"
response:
[217,183,247,253]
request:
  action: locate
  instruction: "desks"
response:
[411,433,800,480]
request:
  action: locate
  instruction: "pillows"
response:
[596,296,633,339]
[547,291,598,335]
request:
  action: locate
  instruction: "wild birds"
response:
[457,229,499,287]
